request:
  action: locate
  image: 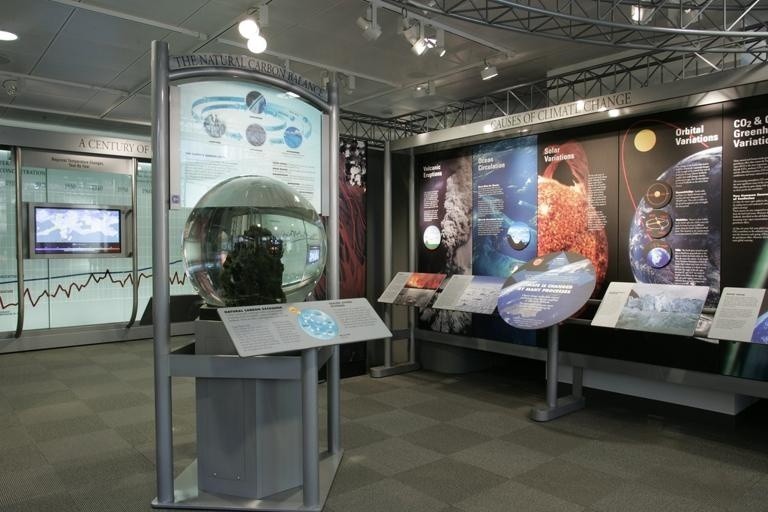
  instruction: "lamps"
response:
[356,13,446,58]
[480,54,499,82]
[630,0,704,31]
[319,70,356,95]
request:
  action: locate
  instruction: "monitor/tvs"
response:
[29,202,126,259]
